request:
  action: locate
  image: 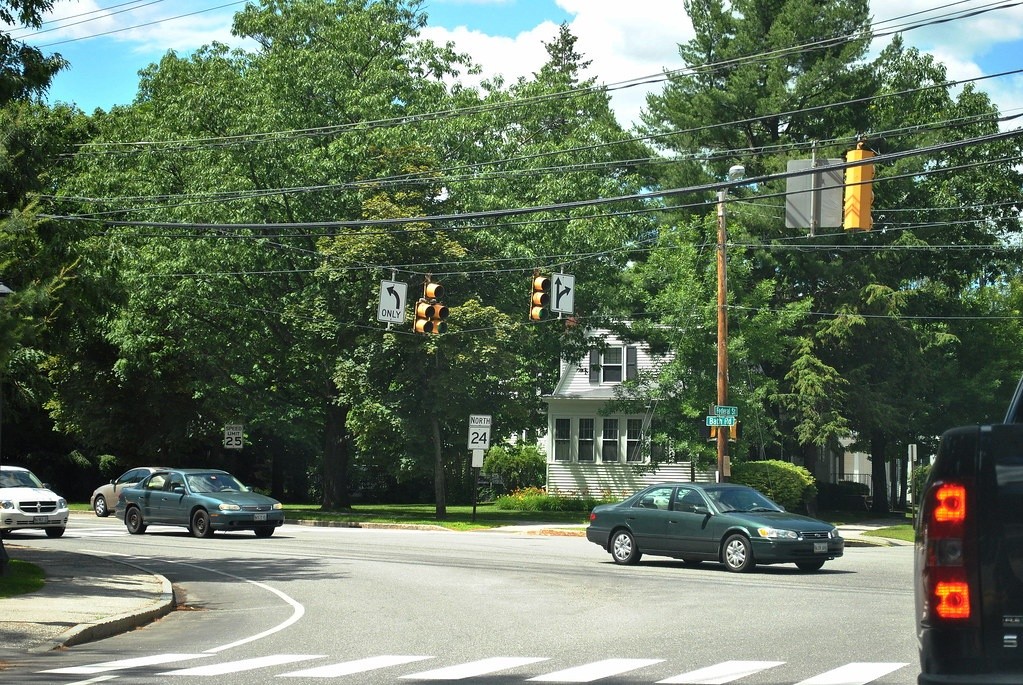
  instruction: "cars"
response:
[1,464,70,539]
[585,480,844,575]
[112,468,285,539]
[90,465,207,519]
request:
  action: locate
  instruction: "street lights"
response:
[712,163,748,483]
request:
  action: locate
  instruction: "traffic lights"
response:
[528,275,552,323]
[842,150,877,233]
[414,282,450,339]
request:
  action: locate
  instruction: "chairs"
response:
[172,483,181,491]
[682,495,696,512]
[642,498,658,509]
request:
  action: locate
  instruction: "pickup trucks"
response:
[909,364,1020,684]
